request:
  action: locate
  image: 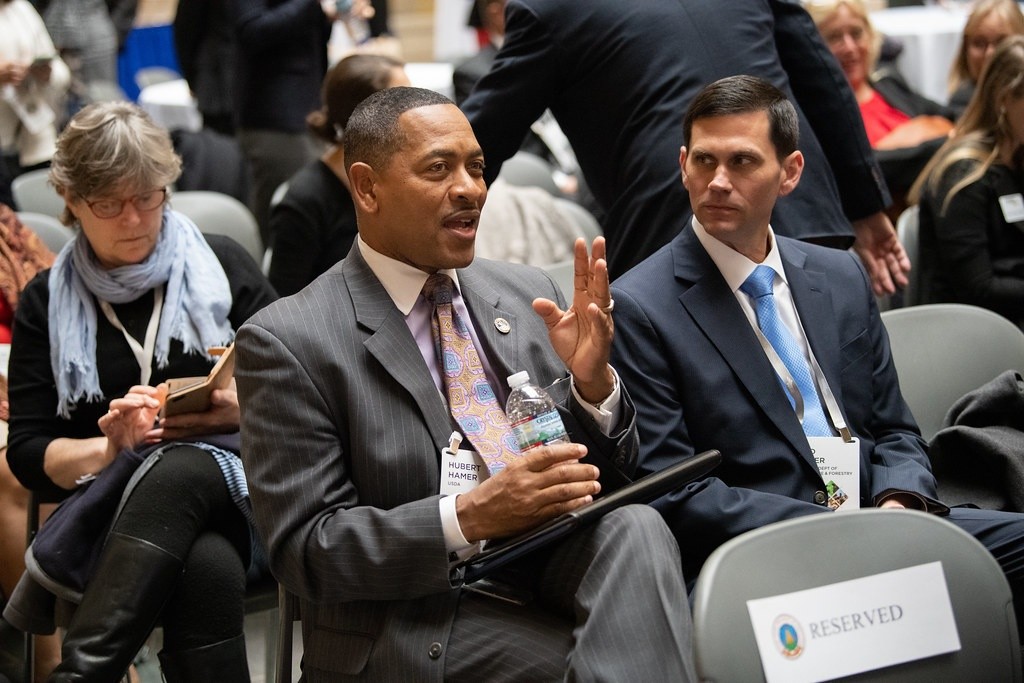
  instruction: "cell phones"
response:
[164,379,211,420]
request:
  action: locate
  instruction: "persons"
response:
[0,1,601,297]
[806,1,1024,331]
[458,1,911,297]
[612,75,1024,616]
[6,101,280,683]
[235,85,698,683]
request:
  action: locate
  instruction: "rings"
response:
[601,299,615,313]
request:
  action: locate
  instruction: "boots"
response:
[45,530,179,682]
[156,631,251,683]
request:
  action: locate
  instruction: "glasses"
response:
[75,181,167,219]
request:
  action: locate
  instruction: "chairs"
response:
[878,303,1024,441]
[693,507,1024,683]
[23,491,279,683]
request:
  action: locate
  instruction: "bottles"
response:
[337,0,371,47]
[505,369,593,509]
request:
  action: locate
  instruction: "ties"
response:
[421,272,520,477]
[741,264,833,437]
